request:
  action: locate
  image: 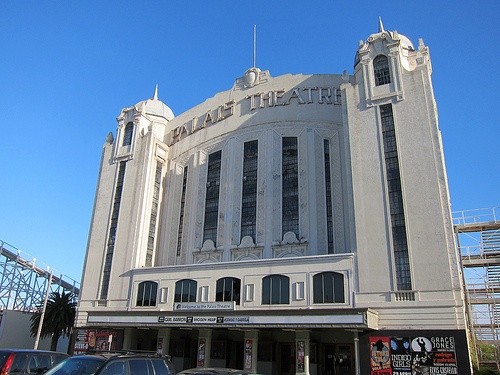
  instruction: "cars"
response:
[176,367,249,375]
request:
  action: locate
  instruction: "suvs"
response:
[0,348,176,375]
